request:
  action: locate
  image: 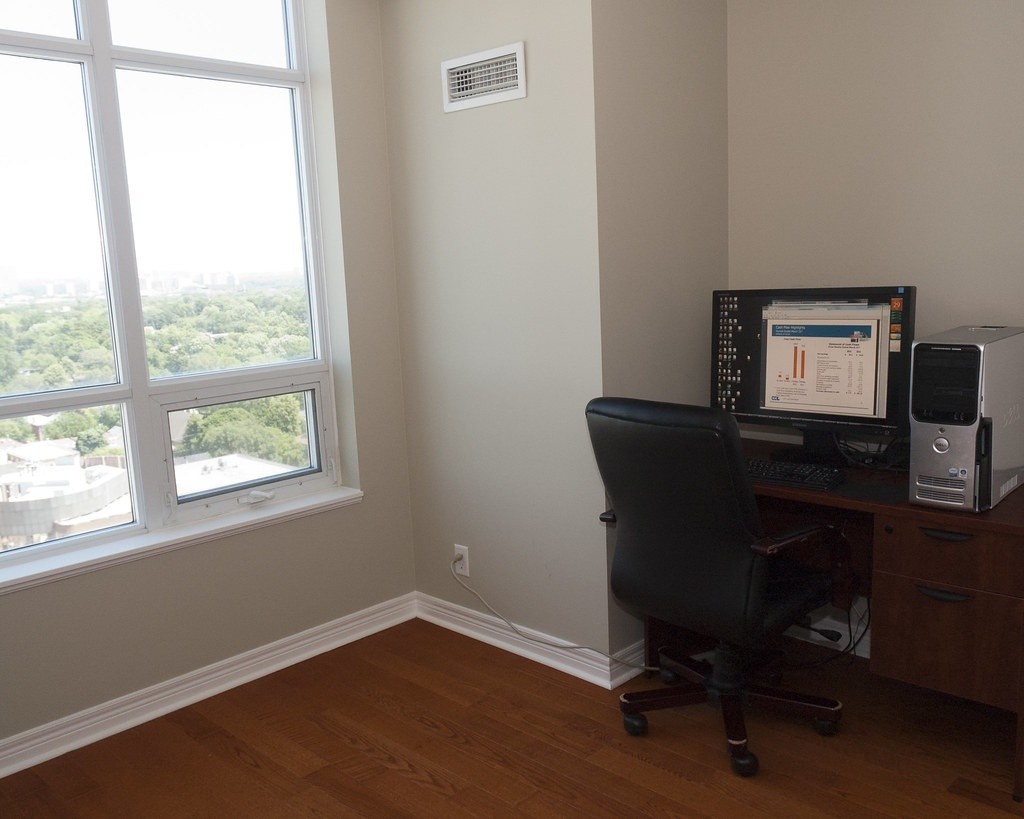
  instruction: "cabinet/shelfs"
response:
[871,514,1024,712]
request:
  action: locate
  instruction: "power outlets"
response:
[454,544,469,578]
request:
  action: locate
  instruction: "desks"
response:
[741,438,1024,536]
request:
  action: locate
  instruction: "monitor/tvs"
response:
[710,285,917,465]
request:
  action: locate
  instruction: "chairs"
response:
[586,398,849,779]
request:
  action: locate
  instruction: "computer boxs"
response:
[907,325,1024,511]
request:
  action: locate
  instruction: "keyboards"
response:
[745,456,847,490]
